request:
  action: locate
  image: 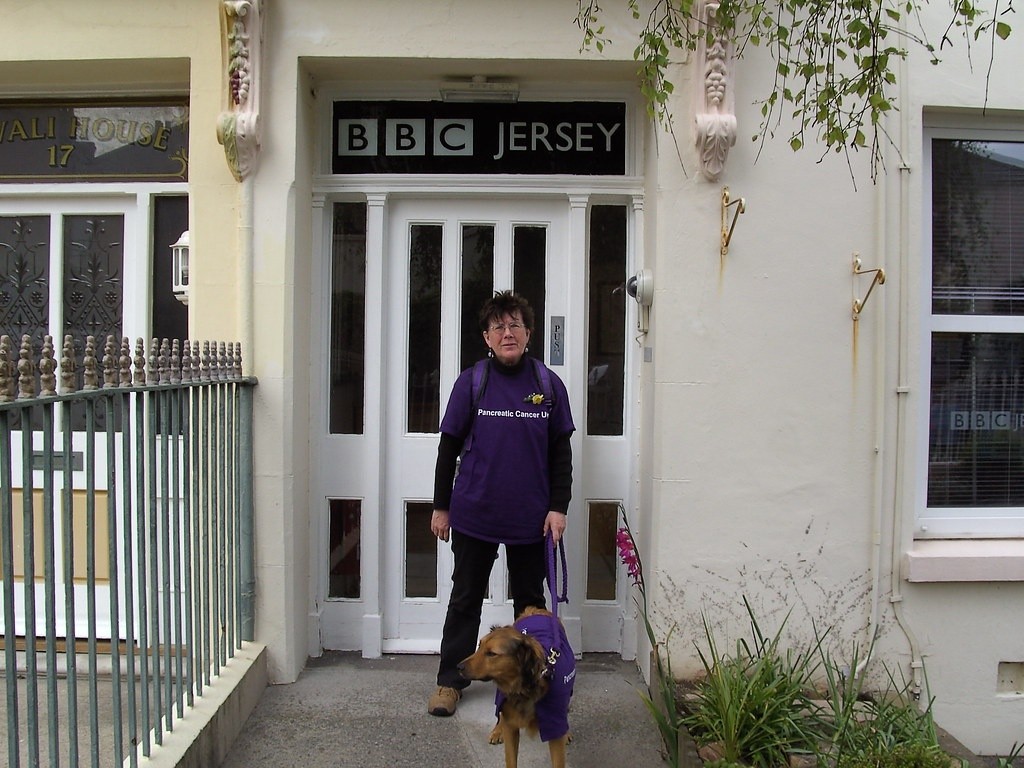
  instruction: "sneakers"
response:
[427,681,462,717]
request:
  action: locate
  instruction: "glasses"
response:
[488,322,525,336]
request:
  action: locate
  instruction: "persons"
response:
[426,288,577,718]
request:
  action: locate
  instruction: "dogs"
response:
[453,603,577,768]
